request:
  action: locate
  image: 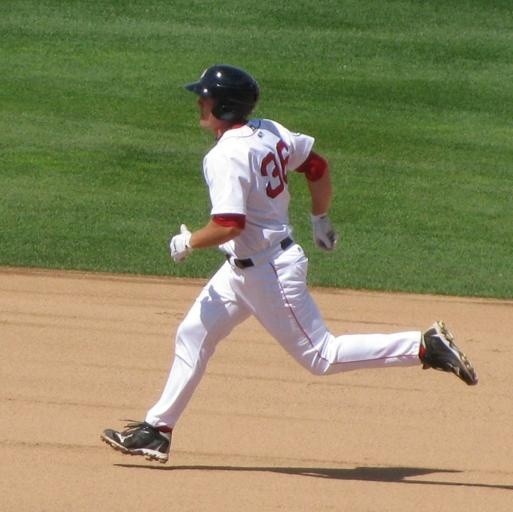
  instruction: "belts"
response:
[234,236,293,269]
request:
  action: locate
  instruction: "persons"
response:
[99,64,479,464]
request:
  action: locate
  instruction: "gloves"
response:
[311,214,339,251]
[169,224,193,264]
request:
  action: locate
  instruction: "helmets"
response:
[184,65,260,122]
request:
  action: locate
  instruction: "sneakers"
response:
[101,421,171,464]
[423,320,478,386]
